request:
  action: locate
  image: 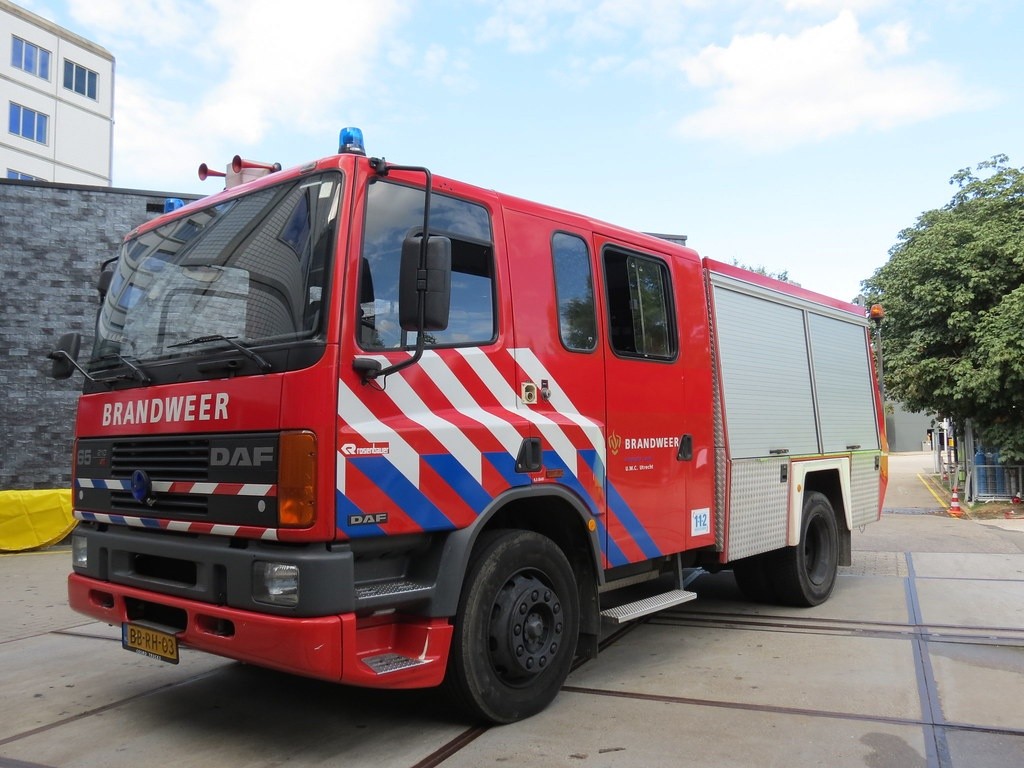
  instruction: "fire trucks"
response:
[47,124,893,725]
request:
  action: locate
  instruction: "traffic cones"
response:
[1012,491,1021,505]
[943,473,948,480]
[947,487,963,513]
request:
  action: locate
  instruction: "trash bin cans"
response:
[922,441,931,451]
